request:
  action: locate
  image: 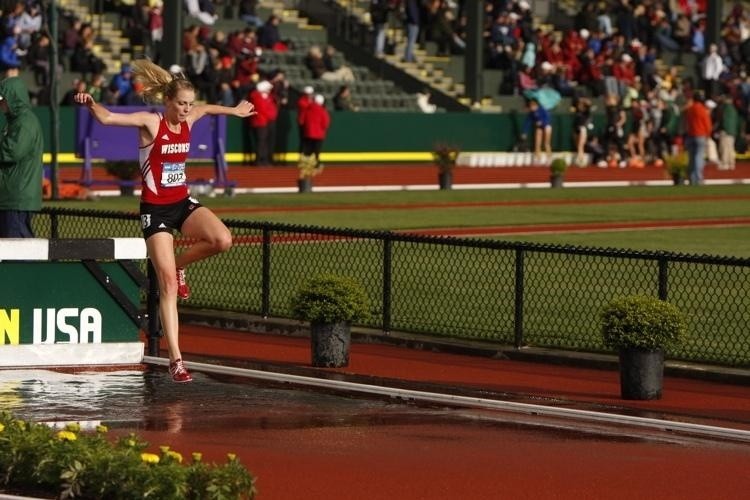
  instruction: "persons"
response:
[0,2,749,188]
[0,76,48,238]
[74,73,260,382]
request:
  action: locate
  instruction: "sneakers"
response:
[177,266,190,300]
[169,359,193,383]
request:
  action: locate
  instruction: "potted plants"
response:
[289,273,370,369]
[430,146,463,189]
[598,293,689,401]
[666,156,688,184]
[106,160,144,197]
[545,157,568,185]
[295,152,324,192]
